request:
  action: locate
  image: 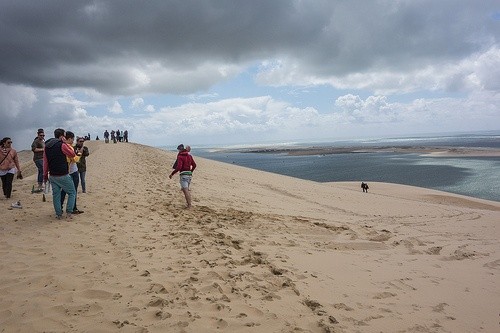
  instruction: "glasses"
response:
[79,141,84,143]
[40,135,45,137]
[6,141,13,144]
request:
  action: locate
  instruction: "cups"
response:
[44,181,50,193]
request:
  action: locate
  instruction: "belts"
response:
[179,173,192,176]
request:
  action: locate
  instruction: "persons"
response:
[43,127,77,220]
[73,137,90,194]
[31,128,47,189]
[361,181,369,193]
[64,131,85,214]
[75,128,129,144]
[168,144,197,210]
[0,137,24,200]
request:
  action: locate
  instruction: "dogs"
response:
[360,182,370,193]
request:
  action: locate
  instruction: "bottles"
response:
[43,194,45,202]
[31,185,34,193]
